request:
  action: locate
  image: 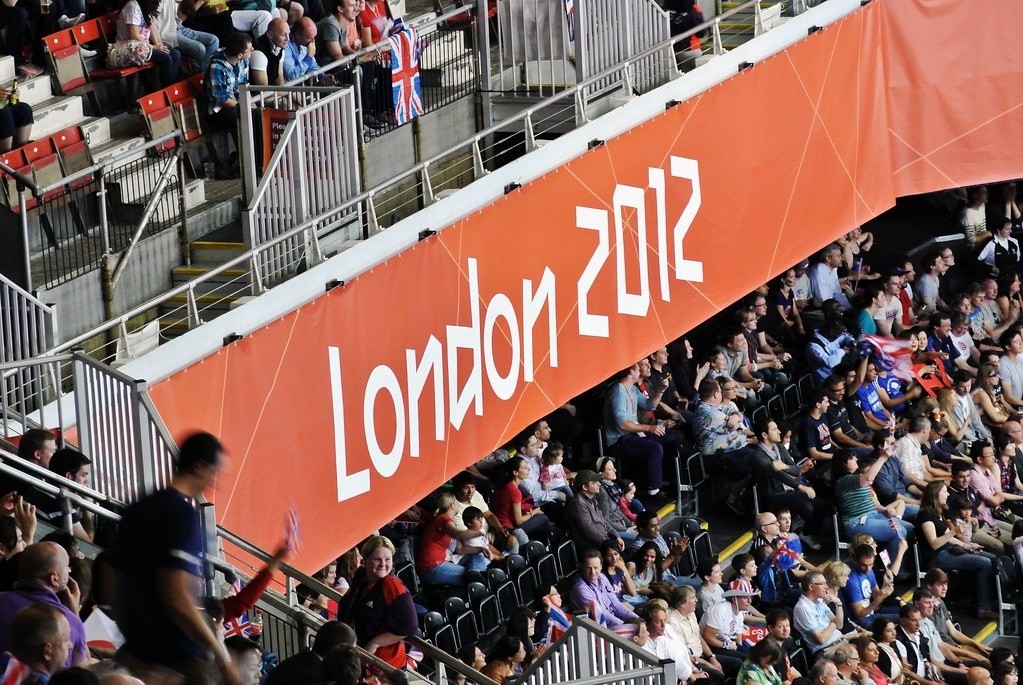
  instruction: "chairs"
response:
[0,0,1017,659]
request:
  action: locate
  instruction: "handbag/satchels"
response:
[106,40,153,69]
[992,507,1015,524]
[983,396,1010,427]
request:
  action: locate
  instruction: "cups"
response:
[204,163,215,182]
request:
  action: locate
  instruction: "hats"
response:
[887,267,911,276]
[722,580,761,597]
[575,470,605,486]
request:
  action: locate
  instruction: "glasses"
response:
[989,371,1000,378]
[942,253,955,258]
[753,302,767,307]
[811,582,827,586]
[849,658,861,661]
[244,47,255,54]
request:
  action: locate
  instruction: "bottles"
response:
[248,609,263,642]
[10,79,19,106]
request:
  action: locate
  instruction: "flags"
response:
[547,599,599,656]
[857,335,914,382]
[388,27,422,126]
[222,576,251,639]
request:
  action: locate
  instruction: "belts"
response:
[824,637,844,648]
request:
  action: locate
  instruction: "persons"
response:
[299,179,1023,685]
[0,86,35,154]
[0,428,360,685]
[0,0,396,172]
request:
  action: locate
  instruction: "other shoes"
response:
[646,491,676,503]
[364,113,388,127]
[977,609,1007,620]
[362,124,380,135]
[81,47,97,58]
[375,109,397,124]
[58,13,86,29]
